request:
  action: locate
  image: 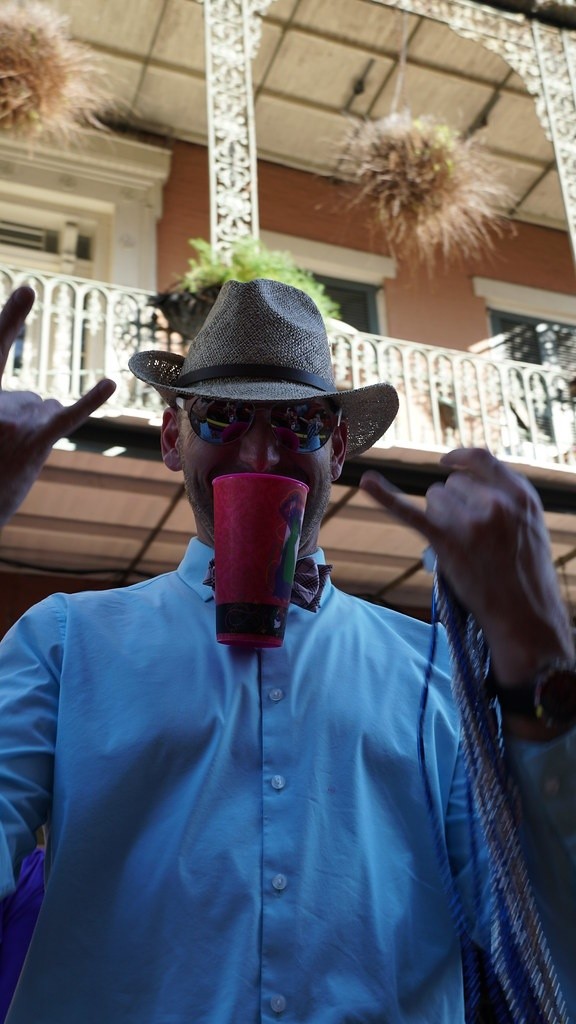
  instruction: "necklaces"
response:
[416,550,567,1024]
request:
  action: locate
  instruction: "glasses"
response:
[174,397,343,454]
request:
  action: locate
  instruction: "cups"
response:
[212,474,309,647]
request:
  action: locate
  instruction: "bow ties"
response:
[201,556,332,613]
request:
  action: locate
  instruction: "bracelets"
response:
[197,418,207,423]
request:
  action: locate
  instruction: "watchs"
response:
[483,660,576,729]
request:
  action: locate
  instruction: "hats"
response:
[129,279,399,460]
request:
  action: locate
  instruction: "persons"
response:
[273,417,299,449]
[307,413,323,450]
[0,280,576,1024]
[220,402,253,443]
[0,847,46,1023]
[286,408,300,434]
[190,398,215,440]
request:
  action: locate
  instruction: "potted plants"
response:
[155,237,338,343]
